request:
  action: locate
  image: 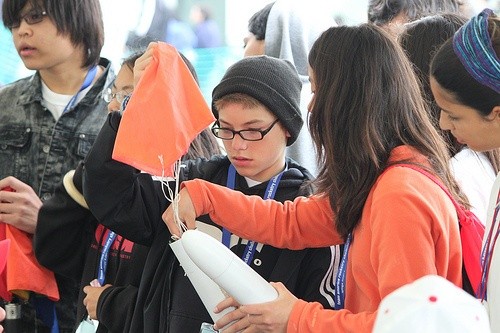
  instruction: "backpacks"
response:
[373,163,485,298]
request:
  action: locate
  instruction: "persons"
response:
[429,9,500,333]
[162,25,471,333]
[242,0,338,179]
[34,49,220,333]
[399,14,496,229]
[82,55,342,333]
[0,0,117,333]
[368,0,462,41]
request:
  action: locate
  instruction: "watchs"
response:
[122,94,130,110]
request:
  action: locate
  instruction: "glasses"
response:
[211,118,279,141]
[103,87,129,102]
[10,8,47,29]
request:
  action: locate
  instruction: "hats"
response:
[211,54,304,146]
